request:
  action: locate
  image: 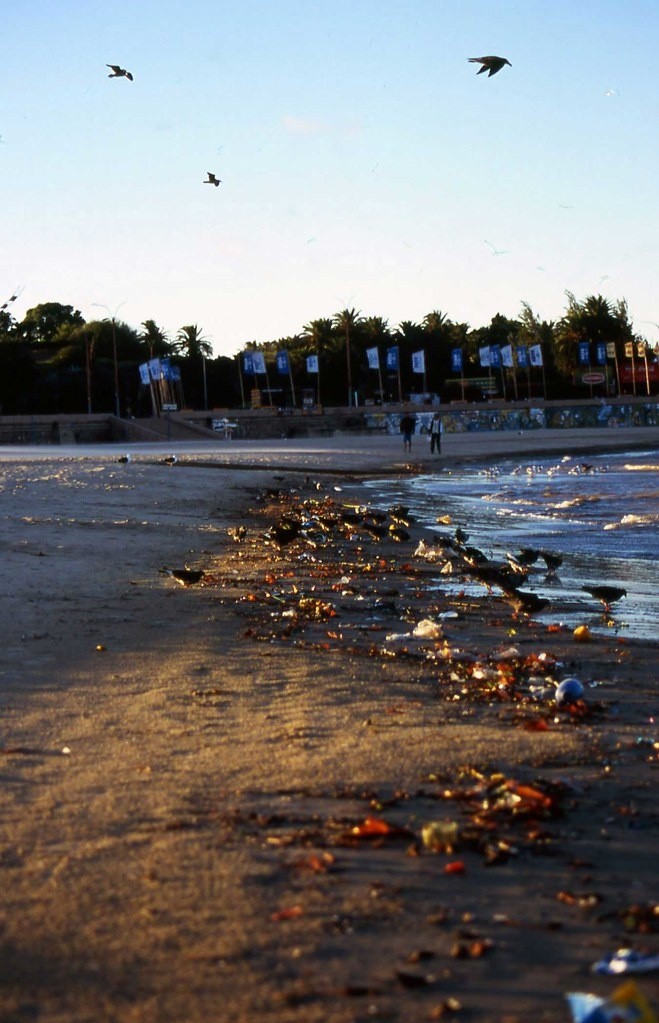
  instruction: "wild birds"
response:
[262,475,628,620]
[161,454,178,466]
[106,64,134,81]
[204,172,222,187]
[116,453,131,468]
[159,567,206,588]
[468,56,512,77]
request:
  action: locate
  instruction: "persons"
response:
[399,412,416,452]
[206,416,213,429]
[428,413,443,455]
